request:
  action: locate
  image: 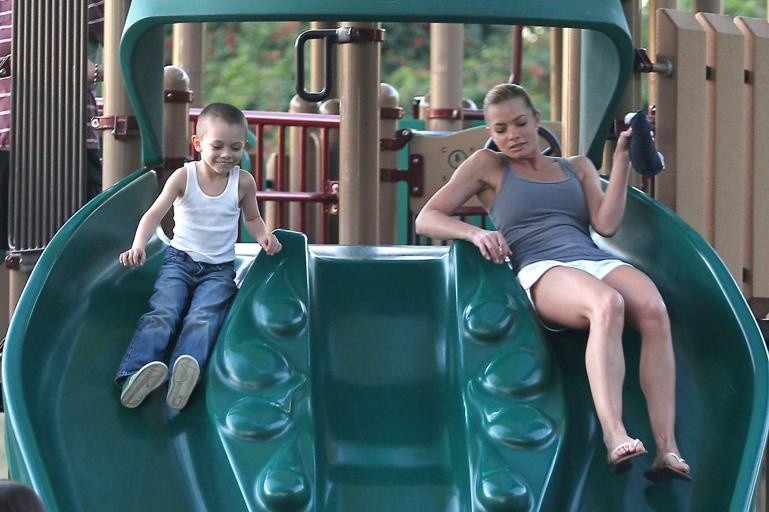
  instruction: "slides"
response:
[1,165,769,512]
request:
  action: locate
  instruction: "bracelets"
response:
[93,62,99,86]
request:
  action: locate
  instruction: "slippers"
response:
[642,453,692,483]
[611,439,648,475]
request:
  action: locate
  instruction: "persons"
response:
[0,0,105,353]
[113,101,283,411]
[415,83,694,483]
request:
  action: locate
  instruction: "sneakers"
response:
[166,355,200,409]
[120,361,168,409]
